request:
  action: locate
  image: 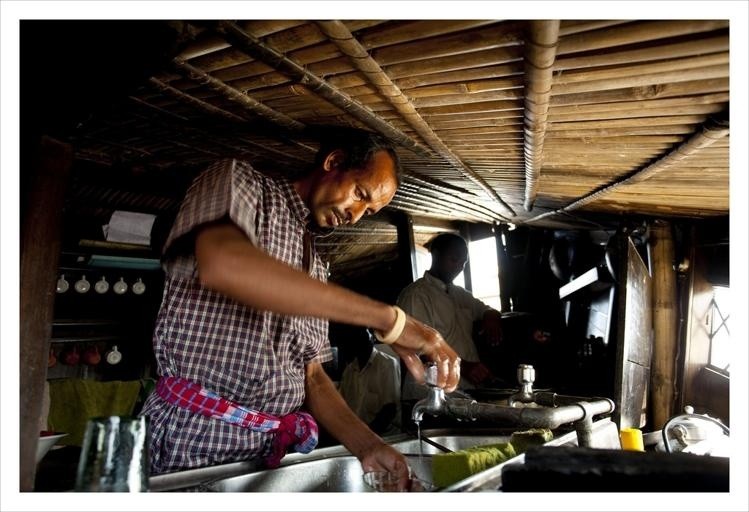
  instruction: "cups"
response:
[76,412,149,492]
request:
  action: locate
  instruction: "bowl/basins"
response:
[35,424,70,467]
[362,469,437,492]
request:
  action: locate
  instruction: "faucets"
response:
[508,364,539,407]
[411,363,448,421]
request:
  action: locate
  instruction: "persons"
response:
[135,127,462,492]
[334,325,405,439]
[394,233,553,431]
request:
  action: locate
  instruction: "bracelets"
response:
[374,305,407,345]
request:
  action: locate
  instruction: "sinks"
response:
[206,455,517,492]
[388,434,515,457]
